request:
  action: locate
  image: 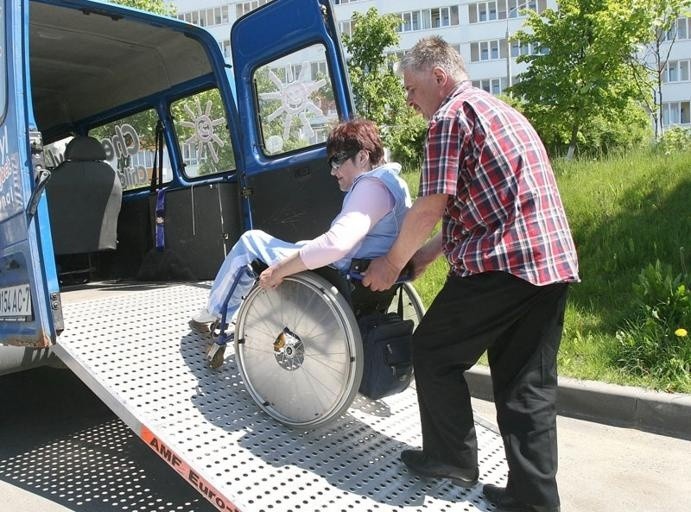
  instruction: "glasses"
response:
[326,153,345,167]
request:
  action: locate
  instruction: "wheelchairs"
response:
[206,256,427,433]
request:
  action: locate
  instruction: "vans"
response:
[1,0,359,379]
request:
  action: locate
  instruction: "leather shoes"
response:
[193,309,231,323]
[483,484,559,510]
[401,450,478,487]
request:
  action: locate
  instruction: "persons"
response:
[357,34,585,512]
[191,114,415,326]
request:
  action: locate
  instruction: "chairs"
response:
[46,137,124,285]
